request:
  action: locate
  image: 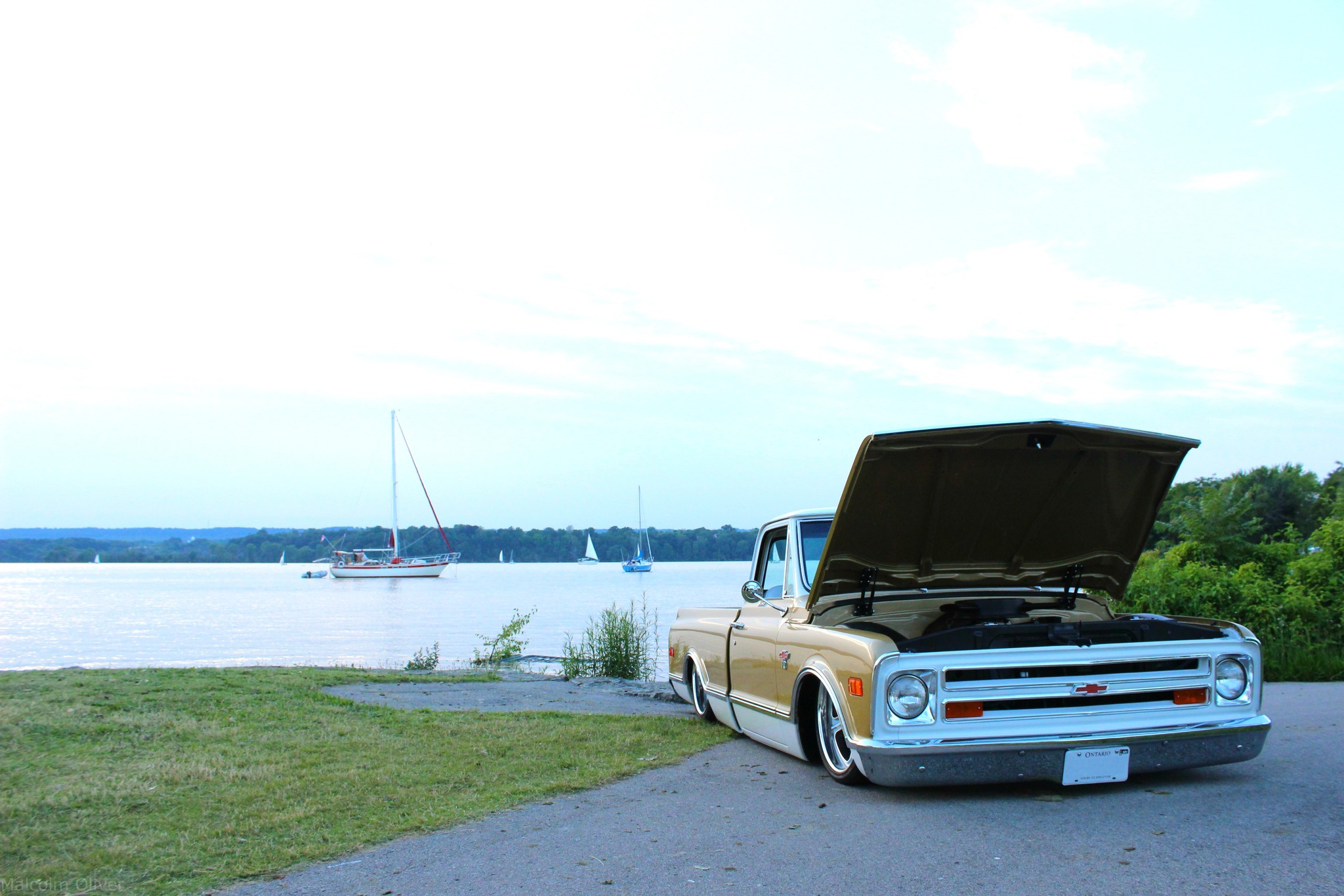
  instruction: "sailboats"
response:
[621,484,653,573]
[576,532,600,565]
[329,409,461,579]
[499,550,504,563]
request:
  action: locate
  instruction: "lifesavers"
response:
[338,561,344,568]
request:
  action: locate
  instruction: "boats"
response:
[301,569,328,579]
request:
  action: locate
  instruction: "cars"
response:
[667,417,1271,795]
